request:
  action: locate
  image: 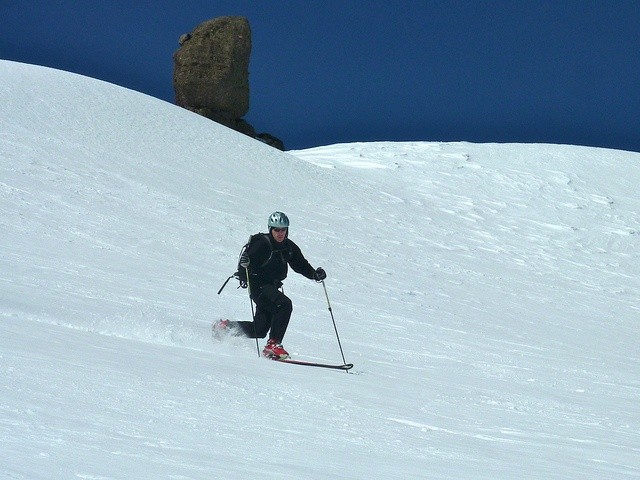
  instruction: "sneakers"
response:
[262,339,289,356]
[217,317,229,329]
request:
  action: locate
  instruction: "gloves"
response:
[240,252,250,267]
[312,267,327,283]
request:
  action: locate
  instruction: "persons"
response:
[212,212,326,359]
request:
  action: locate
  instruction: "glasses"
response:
[274,228,286,232]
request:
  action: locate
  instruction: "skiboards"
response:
[210,319,353,369]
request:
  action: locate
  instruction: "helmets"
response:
[267,211,289,243]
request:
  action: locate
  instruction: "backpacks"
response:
[218,233,293,294]
